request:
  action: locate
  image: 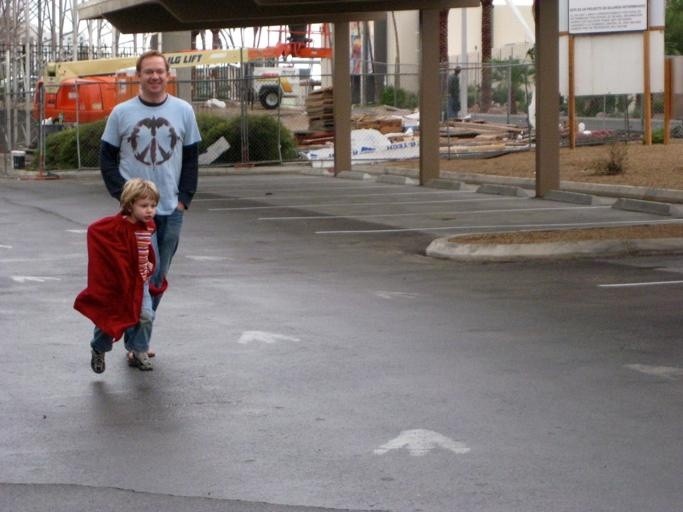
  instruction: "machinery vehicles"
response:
[31,22,332,124]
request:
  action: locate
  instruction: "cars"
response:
[0,76,40,102]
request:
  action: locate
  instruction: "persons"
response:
[97,49,203,358]
[73,177,168,374]
[443,65,462,118]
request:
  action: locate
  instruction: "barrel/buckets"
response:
[11,150,27,168]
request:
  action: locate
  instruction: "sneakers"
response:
[133,352,152,371]
[91,350,105,373]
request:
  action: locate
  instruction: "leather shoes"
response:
[128,352,134,361]
[147,351,154,357]
